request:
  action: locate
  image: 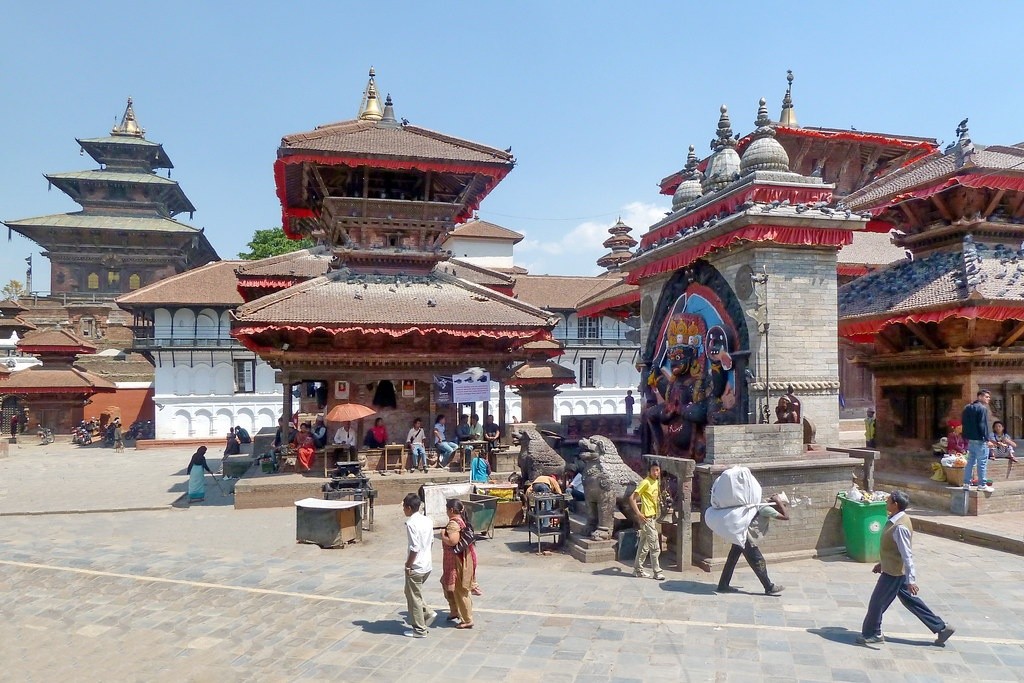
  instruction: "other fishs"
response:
[290,246,467,306]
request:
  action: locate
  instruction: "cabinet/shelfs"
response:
[527,493,565,554]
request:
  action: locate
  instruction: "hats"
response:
[866,406,876,413]
[946,419,962,429]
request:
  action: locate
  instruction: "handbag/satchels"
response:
[406,436,415,449]
[453,526,476,554]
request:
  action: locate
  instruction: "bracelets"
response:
[404,563,411,572]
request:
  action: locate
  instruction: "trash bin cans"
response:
[836,491,888,562]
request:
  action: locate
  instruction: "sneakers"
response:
[653,573,665,580]
[857,633,885,644]
[963,484,969,490]
[632,571,651,578]
[977,484,994,493]
[934,623,956,645]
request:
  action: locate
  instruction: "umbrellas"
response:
[326,403,376,422]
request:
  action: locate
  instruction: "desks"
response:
[295,498,366,547]
[324,444,351,478]
[384,443,405,471]
[460,441,489,474]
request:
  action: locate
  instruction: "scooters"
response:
[124,416,155,441]
[71,416,100,446]
[37,424,55,445]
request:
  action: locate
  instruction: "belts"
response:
[646,514,657,520]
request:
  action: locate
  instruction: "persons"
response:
[531,465,585,528]
[270,414,327,473]
[362,418,388,450]
[334,421,356,467]
[864,408,875,447]
[716,493,789,595]
[114,424,123,453]
[456,414,500,482]
[10,414,18,438]
[947,388,1018,492]
[187,446,213,504]
[440,499,482,628]
[625,390,635,428]
[217,426,252,473]
[434,414,458,468]
[629,460,665,580]
[403,493,438,638]
[855,490,957,644]
[406,418,428,471]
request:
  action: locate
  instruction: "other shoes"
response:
[490,447,500,452]
[307,468,311,472]
[446,611,460,621]
[1009,457,1018,463]
[438,462,443,467]
[410,466,416,471]
[456,622,474,629]
[362,445,369,450]
[404,630,427,638]
[989,453,996,460]
[423,467,428,471]
[424,610,437,621]
[470,589,482,595]
[766,585,785,595]
[717,586,738,592]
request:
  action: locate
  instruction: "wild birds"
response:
[637,125,872,257]
[837,242,1024,311]
[945,141,957,150]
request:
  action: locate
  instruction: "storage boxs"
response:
[262,462,272,472]
